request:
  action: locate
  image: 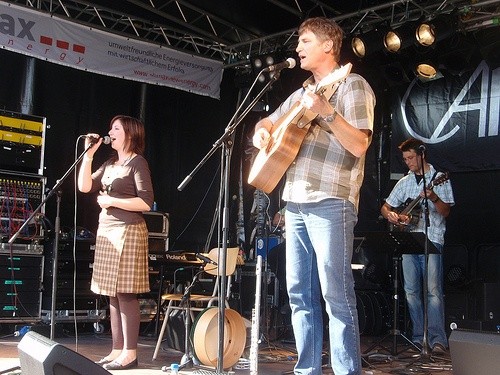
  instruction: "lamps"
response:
[250,50,287,88]
[351,20,393,58]
[382,13,427,54]
[414,41,447,79]
[415,10,458,47]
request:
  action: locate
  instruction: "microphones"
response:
[262,57,297,72]
[416,145,426,154]
[79,135,110,145]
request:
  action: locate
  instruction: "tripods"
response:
[354,230,441,362]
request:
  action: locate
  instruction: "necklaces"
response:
[121,155,131,165]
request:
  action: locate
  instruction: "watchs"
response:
[322,111,337,124]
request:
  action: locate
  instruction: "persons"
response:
[273,204,286,239]
[77,114,154,369]
[381,138,455,355]
[251,17,377,375]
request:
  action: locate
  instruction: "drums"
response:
[190,306,247,369]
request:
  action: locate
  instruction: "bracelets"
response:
[432,196,439,203]
[84,156,93,161]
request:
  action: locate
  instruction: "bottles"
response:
[14,326,32,337]
[170,364,179,375]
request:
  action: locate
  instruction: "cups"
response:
[151,201,156,212]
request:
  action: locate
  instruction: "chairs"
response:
[151,245,241,367]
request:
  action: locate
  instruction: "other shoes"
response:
[407,343,423,353]
[96,358,112,366]
[103,358,138,369]
[432,344,445,354]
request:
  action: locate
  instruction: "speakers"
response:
[16,330,114,375]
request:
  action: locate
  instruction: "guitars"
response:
[245,63,352,195]
[387,172,449,232]
